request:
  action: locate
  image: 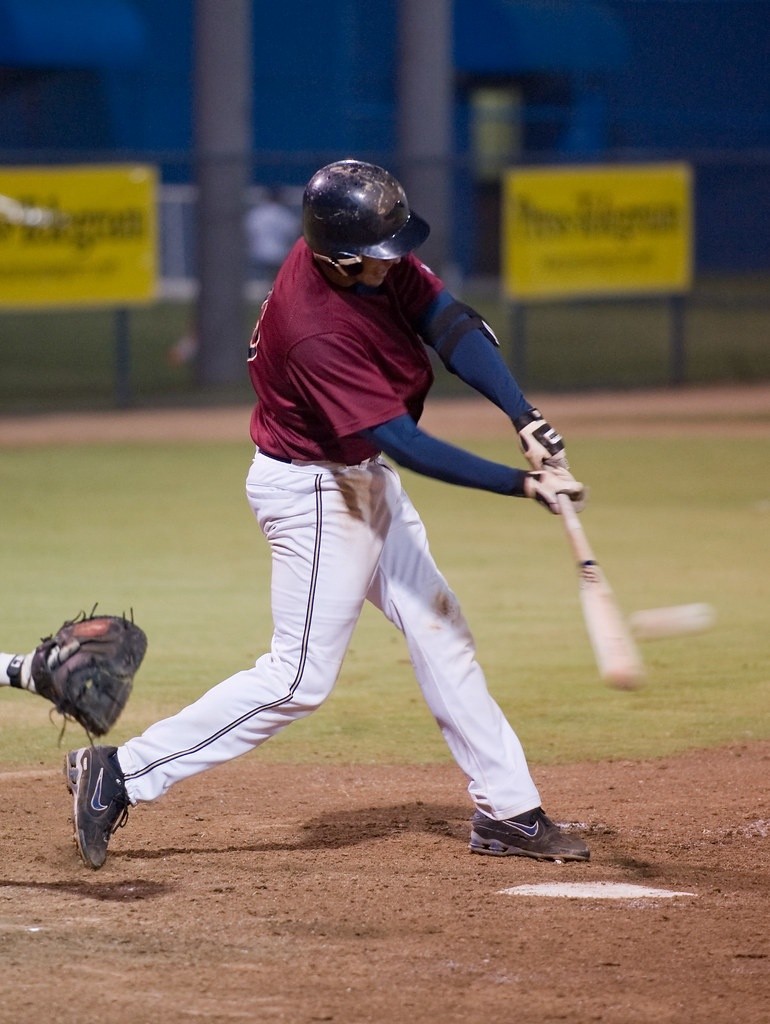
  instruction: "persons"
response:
[243,182,303,282]
[0,601,148,740]
[64,160,590,872]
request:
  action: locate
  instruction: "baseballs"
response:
[676,603,715,634]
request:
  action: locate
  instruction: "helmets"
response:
[302,159,430,277]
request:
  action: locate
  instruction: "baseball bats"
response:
[544,464,644,692]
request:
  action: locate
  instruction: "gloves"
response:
[518,468,586,515]
[512,408,570,473]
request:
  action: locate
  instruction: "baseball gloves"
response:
[29,600,150,758]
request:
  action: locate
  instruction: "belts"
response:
[258,448,362,466]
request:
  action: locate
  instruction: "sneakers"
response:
[64,744,131,871]
[469,807,590,863]
